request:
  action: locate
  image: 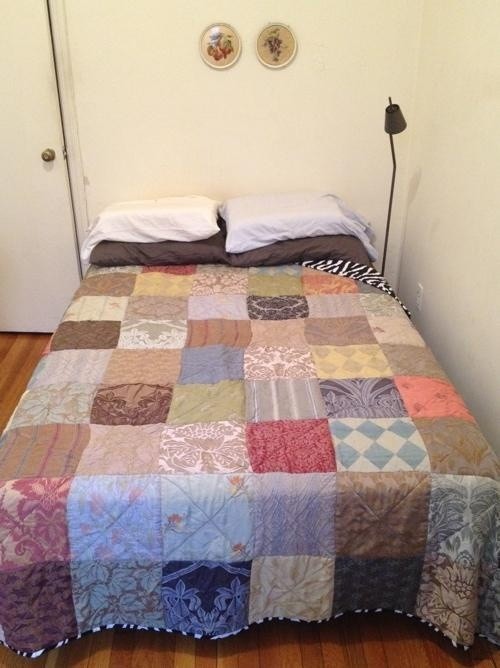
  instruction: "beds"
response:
[0,195,500,659]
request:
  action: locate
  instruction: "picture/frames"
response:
[198,22,241,69]
[255,22,297,69]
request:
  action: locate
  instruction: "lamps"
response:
[381,96,407,276]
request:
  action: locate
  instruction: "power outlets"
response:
[415,282,423,311]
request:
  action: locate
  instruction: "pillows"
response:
[219,191,378,262]
[81,195,223,266]
